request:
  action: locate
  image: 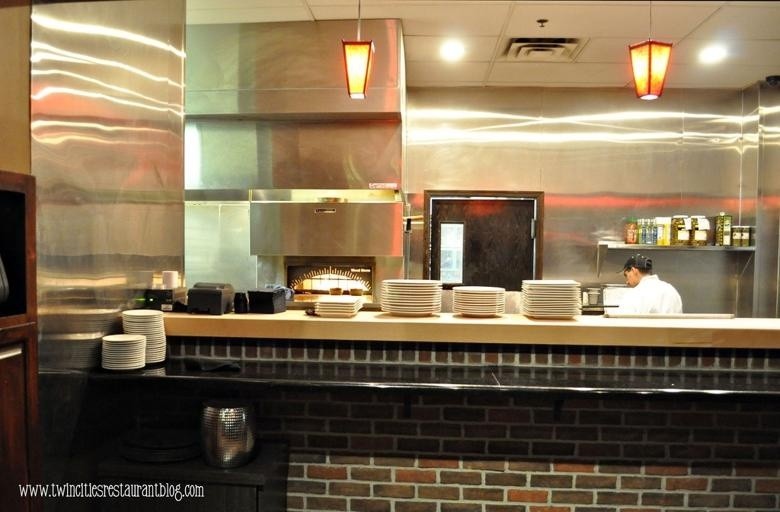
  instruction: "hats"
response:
[616,253,652,273]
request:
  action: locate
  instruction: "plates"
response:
[313,278,584,321]
[99,308,168,373]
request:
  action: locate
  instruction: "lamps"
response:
[627,0,675,102]
[339,0,376,101]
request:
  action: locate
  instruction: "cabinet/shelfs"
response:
[56,442,288,512]
[596,237,756,280]
[0,169,44,512]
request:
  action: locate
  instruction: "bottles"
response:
[626,212,752,247]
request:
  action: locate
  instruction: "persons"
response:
[614,254,684,314]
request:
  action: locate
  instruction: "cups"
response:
[161,269,179,289]
[587,287,600,305]
